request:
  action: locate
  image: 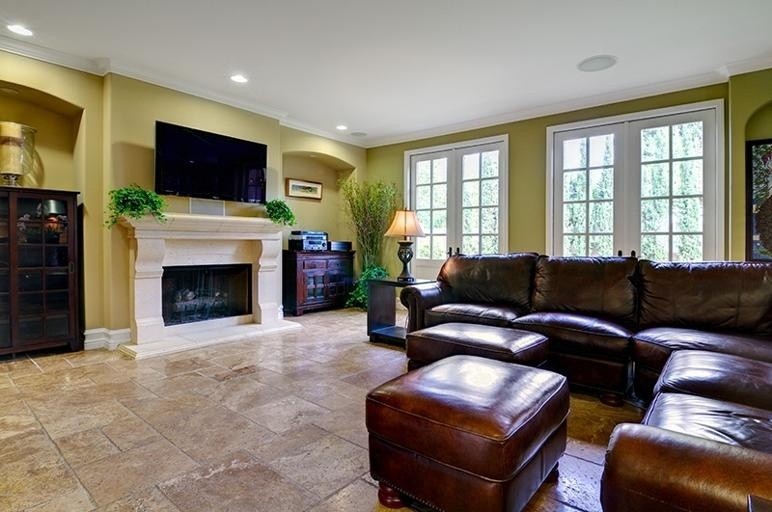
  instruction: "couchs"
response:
[399,251,771,511]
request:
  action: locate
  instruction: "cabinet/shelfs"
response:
[1,186,87,361]
[282,249,358,316]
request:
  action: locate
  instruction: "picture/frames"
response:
[286,178,323,201]
[743,138,771,263]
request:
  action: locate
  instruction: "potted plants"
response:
[102,182,168,229]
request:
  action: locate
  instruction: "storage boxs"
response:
[327,240,352,250]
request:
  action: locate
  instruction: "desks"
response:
[365,277,437,353]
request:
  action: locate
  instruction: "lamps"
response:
[383,207,427,282]
[1,119,39,188]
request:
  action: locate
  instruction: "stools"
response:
[402,321,553,372]
[360,353,571,511]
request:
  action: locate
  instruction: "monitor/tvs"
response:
[155,120,268,206]
